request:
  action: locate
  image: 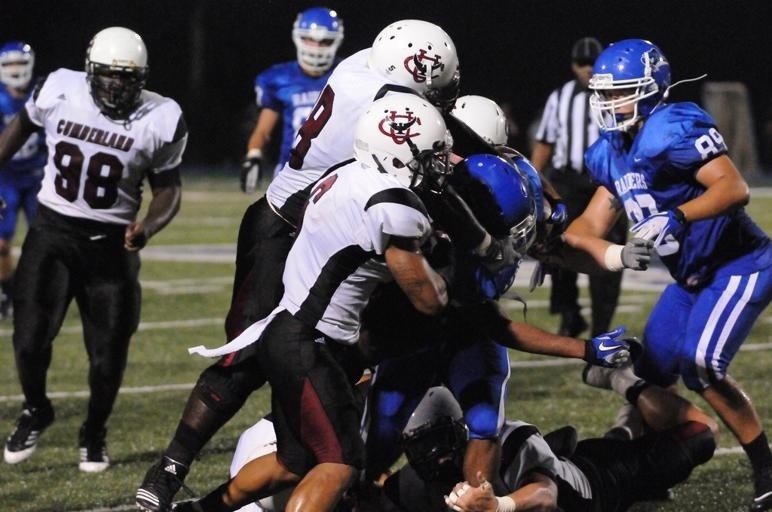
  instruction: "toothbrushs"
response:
[542,199,555,240]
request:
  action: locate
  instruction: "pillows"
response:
[0,42,35,87]
[448,154,538,257]
[449,94,508,146]
[514,157,543,222]
[291,6,345,71]
[368,18,461,113]
[402,386,469,484]
[588,38,671,134]
[354,94,454,202]
[85,25,151,119]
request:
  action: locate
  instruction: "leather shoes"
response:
[628,207,688,249]
[620,238,653,271]
[546,199,569,224]
[239,148,263,193]
[585,325,631,369]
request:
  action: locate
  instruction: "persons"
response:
[556,38,772,511]
[0,23,189,476]
[150,20,641,512]
[369,336,719,512]
[240,8,344,196]
[529,37,634,385]
[0,33,50,316]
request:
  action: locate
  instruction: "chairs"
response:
[136,455,190,512]
[78,420,111,473]
[557,312,588,338]
[750,465,772,511]
[617,404,645,436]
[3,399,54,464]
[582,336,646,390]
